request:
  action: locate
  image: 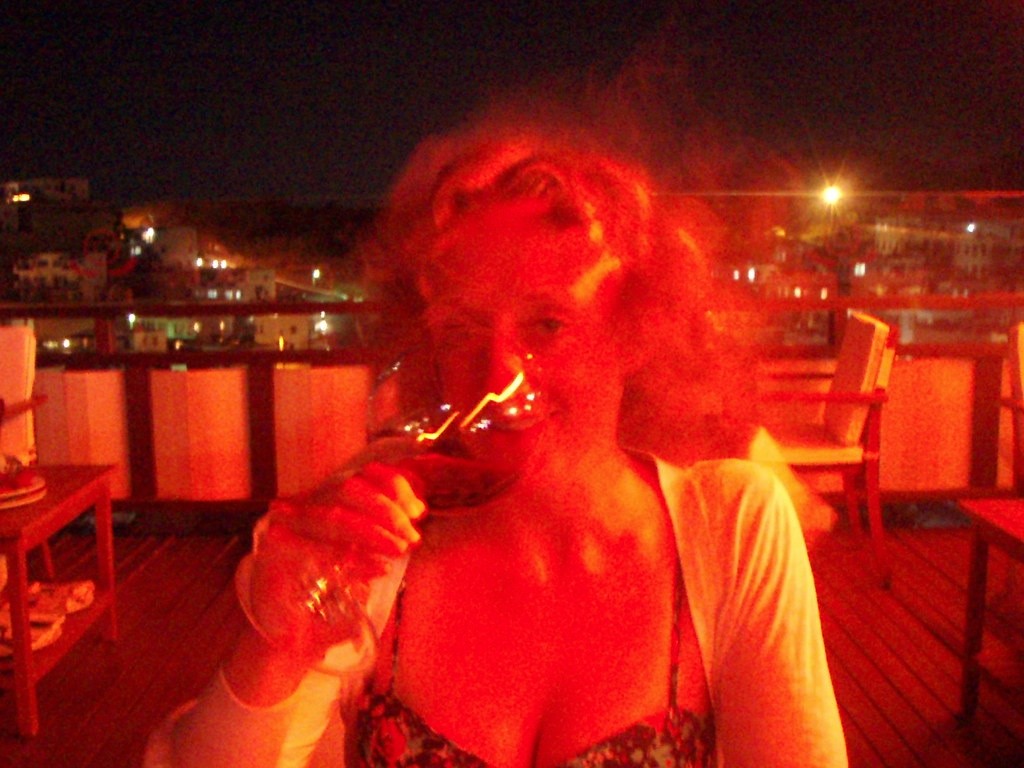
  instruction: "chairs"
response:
[0,323,55,580]
[951,328,1024,735]
[746,306,890,589]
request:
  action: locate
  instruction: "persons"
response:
[141,114,848,767]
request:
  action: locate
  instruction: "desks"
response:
[0,458,117,738]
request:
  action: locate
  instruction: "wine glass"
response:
[232,322,549,679]
